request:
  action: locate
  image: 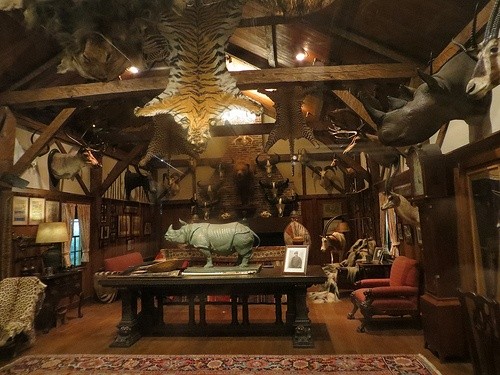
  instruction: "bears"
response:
[231,161,250,178]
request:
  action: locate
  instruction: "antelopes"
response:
[319,211,347,264]
[465,0,500,100]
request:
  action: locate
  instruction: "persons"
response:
[291,251,301,268]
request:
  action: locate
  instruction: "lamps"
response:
[34,222,69,277]
[296,48,305,60]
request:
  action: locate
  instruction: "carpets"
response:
[0,342,442,375]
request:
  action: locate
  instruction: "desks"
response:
[98,264,327,348]
[27,270,84,335]
[151,246,290,304]
[357,261,393,280]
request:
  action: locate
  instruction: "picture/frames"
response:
[44,201,59,223]
[28,197,46,226]
[101,205,152,251]
[283,244,310,275]
[12,196,29,225]
[397,223,422,244]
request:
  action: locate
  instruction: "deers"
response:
[48,123,109,181]
[326,108,409,159]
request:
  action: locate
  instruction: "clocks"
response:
[407,142,468,365]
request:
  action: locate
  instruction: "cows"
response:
[264,193,300,219]
[257,175,290,202]
[209,157,232,181]
[188,195,222,221]
[195,178,224,200]
[255,152,281,178]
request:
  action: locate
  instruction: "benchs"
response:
[95,253,146,303]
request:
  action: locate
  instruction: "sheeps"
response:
[160,171,182,197]
[376,161,421,227]
[310,164,337,189]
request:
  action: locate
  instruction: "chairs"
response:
[347,256,419,334]
[458,289,500,374]
[0,277,47,361]
[322,237,379,300]
